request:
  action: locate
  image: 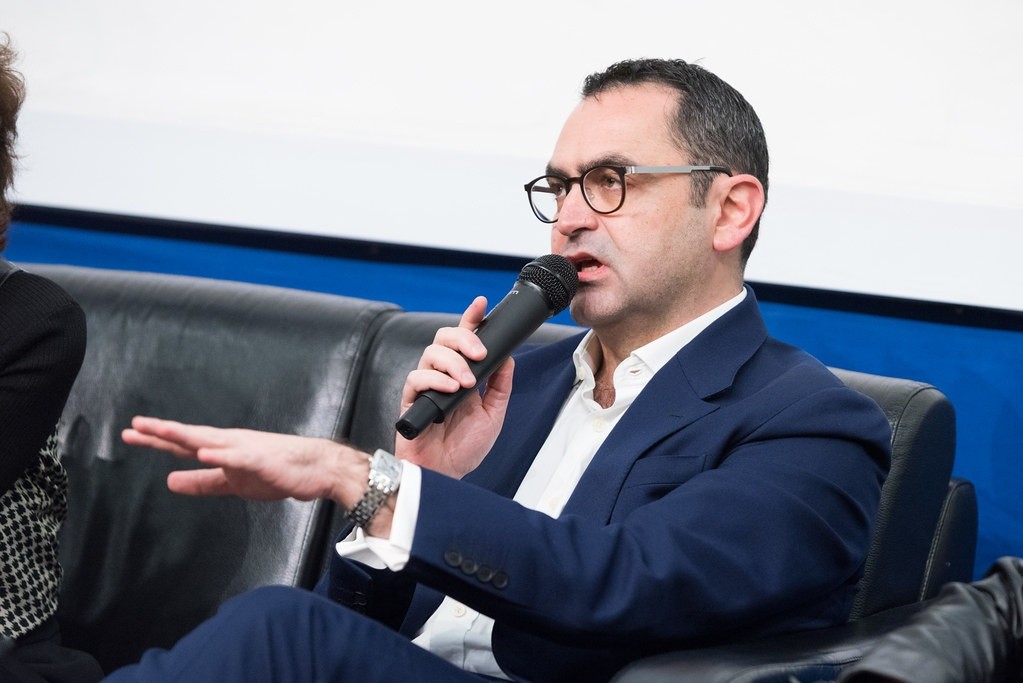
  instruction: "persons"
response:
[840,549,1022,683]
[3,34,86,682]
[91,59,889,683]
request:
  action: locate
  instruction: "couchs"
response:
[0,252,981,683]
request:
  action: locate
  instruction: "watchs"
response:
[347,440,402,531]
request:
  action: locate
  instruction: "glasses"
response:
[523,159,733,227]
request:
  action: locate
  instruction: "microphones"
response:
[395,254,579,441]
